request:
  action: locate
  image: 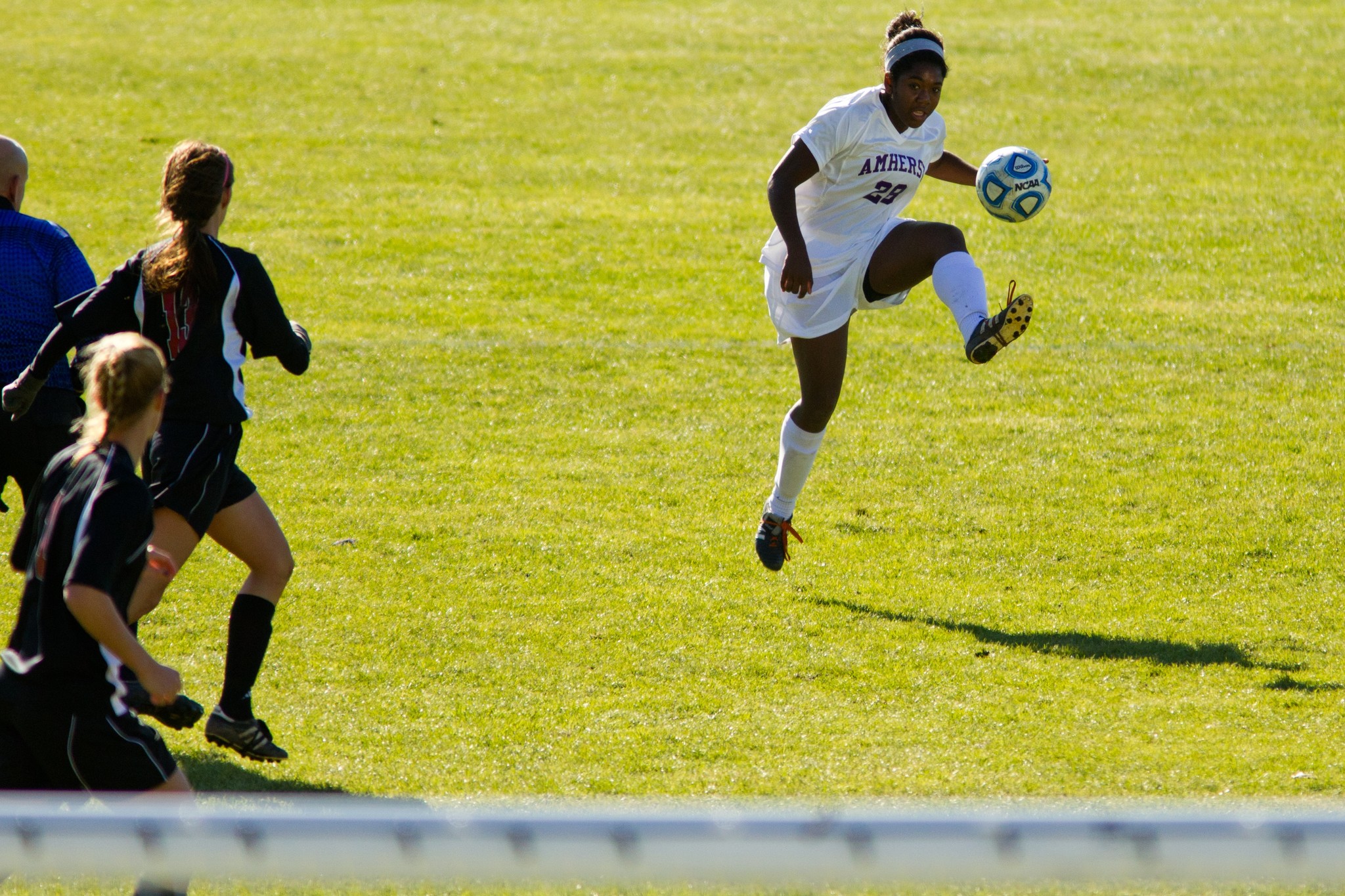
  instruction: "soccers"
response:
[975,146,1053,223]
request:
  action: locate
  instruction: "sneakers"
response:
[205,704,288,763]
[121,680,205,729]
[754,496,792,571]
[965,280,1034,366]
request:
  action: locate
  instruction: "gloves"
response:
[1,365,49,421]
[289,319,312,350]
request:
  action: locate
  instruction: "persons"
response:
[2,141,312,766]
[0,133,98,515]
[756,11,1048,574]
[0,331,196,896]
